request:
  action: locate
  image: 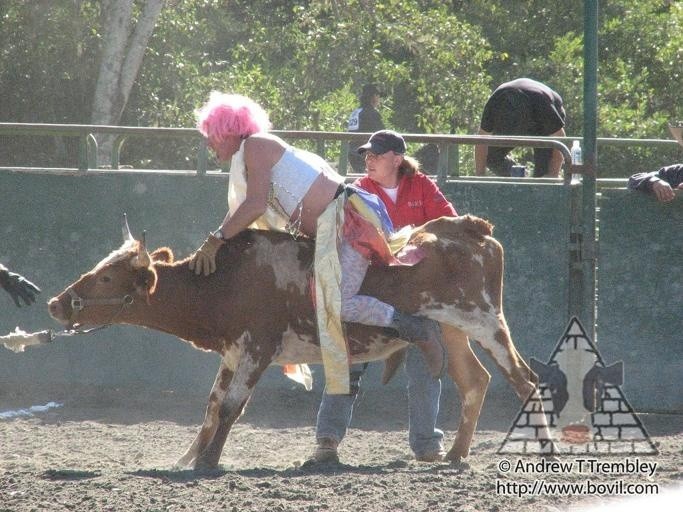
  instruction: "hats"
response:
[356,130,406,155]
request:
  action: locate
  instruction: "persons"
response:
[626,163,683,202]
[187,92,450,381]
[0,263,42,309]
[474,77,567,178]
[346,83,440,175]
[301,128,460,471]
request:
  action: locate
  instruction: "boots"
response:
[391,310,448,380]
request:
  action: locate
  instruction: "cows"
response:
[48,211,561,474]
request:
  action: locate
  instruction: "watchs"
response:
[213,230,224,240]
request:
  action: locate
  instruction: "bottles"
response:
[570,140,582,181]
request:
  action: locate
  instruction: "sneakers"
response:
[317,438,339,462]
[417,449,446,463]
